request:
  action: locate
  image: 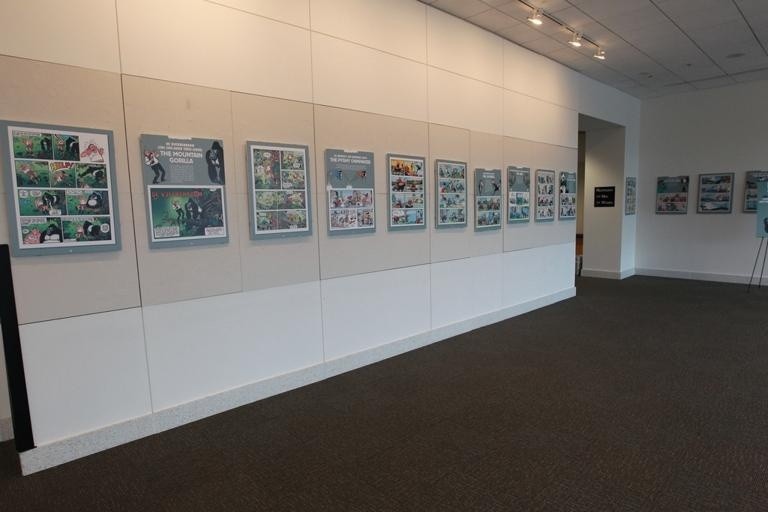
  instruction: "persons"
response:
[171,189,224,233]
[206,141,224,185]
[145,150,166,184]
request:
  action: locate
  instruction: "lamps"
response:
[527,6,606,61]
